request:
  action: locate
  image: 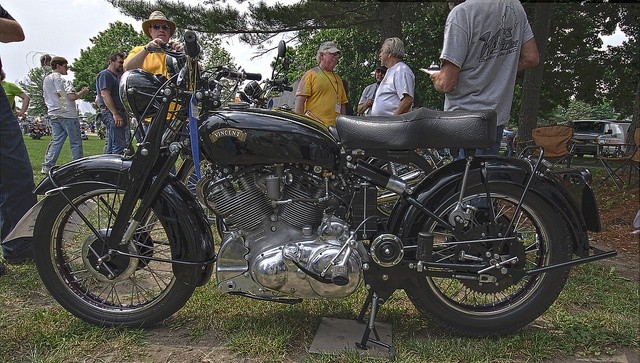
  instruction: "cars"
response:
[567,120,624,157]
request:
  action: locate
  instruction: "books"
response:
[419,60,441,75]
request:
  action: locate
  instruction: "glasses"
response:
[150,23,174,33]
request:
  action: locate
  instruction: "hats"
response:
[141,10,177,40]
[318,40,344,57]
[375,59,387,72]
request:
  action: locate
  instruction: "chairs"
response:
[597,127,639,190]
[529,126,576,174]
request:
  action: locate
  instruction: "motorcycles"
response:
[30,31,617,358]
[187,40,447,225]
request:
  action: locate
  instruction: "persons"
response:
[122,10,201,197]
[95,51,127,155]
[294,41,349,128]
[429,0,539,209]
[356,60,389,114]
[0,4,38,276]
[40,56,90,175]
[371,37,416,115]
[0,57,30,119]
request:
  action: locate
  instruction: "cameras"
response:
[156,42,174,52]
